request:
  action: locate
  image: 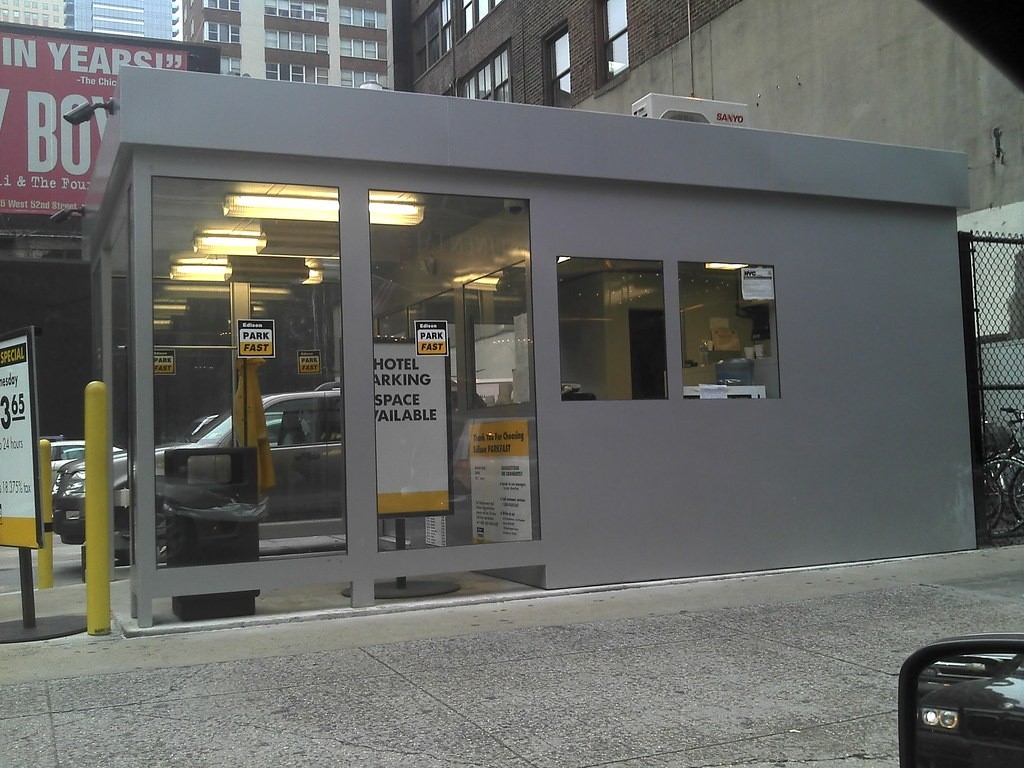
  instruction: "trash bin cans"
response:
[162,445,271,622]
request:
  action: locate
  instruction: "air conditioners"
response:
[632,93,749,129]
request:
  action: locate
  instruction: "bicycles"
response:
[977,405,1024,539]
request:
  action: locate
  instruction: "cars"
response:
[919,654,1024,768]
[48,433,122,496]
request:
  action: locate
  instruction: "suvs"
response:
[49,381,343,566]
